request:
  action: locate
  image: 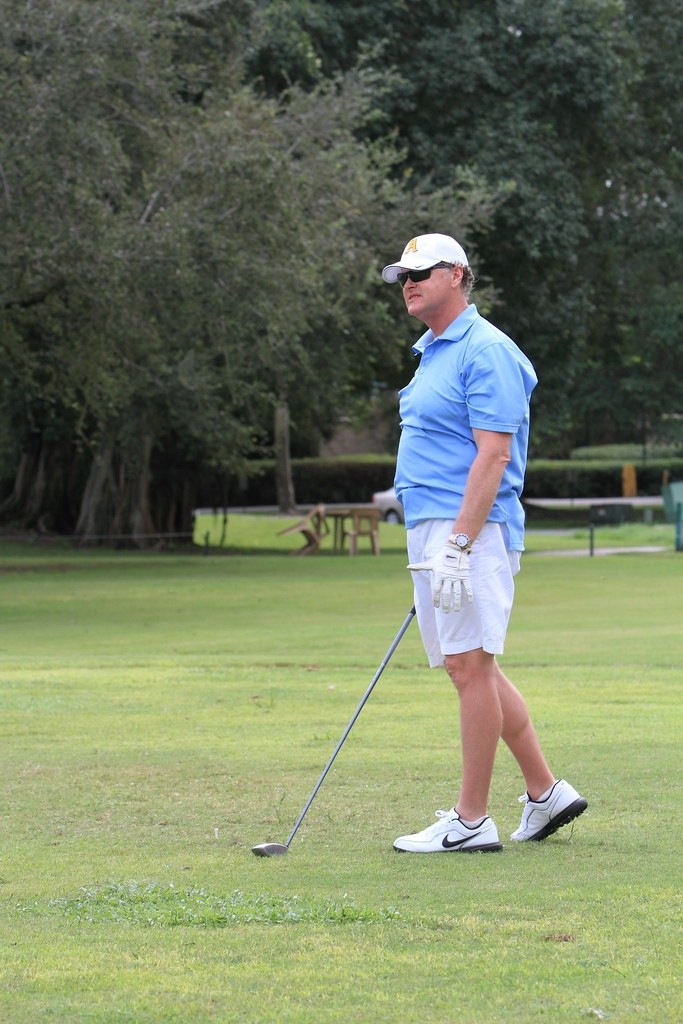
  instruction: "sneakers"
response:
[393,807,503,854]
[510,778,588,843]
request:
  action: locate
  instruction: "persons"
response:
[380,231,588,852]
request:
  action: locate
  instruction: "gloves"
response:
[407,543,473,613]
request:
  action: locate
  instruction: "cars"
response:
[373,484,407,523]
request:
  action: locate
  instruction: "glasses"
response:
[397,265,451,288]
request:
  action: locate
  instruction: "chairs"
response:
[277,503,331,556]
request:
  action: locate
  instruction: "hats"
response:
[382,233,468,283]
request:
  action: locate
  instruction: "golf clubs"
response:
[251,606,418,858]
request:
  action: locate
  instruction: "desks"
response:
[315,503,381,558]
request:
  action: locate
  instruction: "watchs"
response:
[448,533,471,549]
